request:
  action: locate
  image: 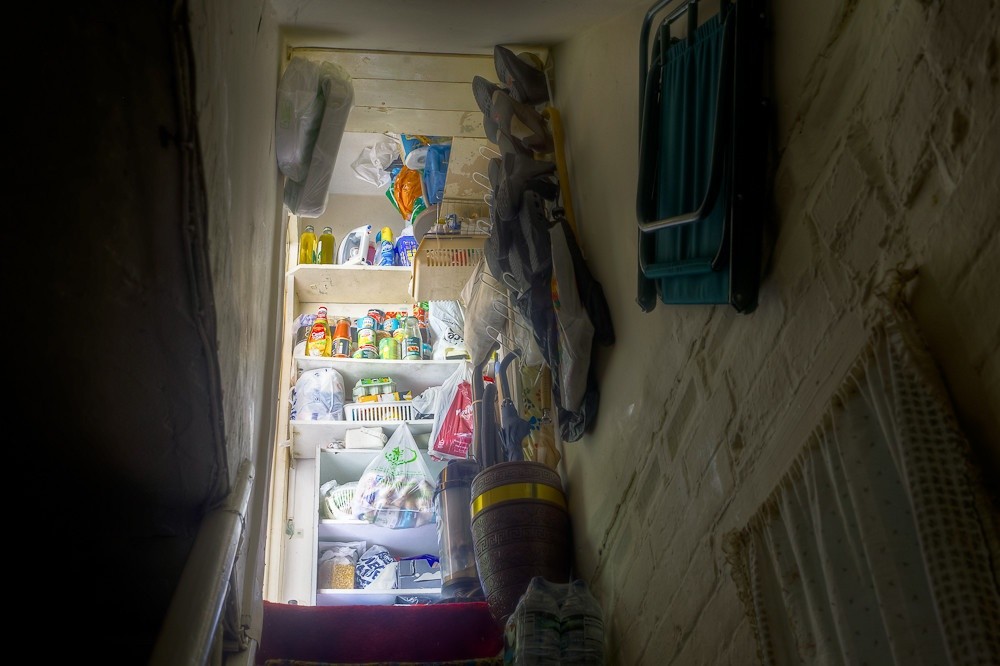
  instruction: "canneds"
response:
[353,315,407,361]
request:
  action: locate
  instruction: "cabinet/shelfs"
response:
[310,447,444,605]
[289,264,470,460]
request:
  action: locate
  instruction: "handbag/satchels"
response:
[546,222,595,412]
[427,359,475,460]
[460,255,508,368]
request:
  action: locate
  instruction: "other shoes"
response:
[471,45,561,370]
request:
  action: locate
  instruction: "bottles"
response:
[314,227,335,264]
[299,224,317,264]
[304,305,332,357]
[514,574,605,666]
[401,315,422,360]
[331,318,352,357]
